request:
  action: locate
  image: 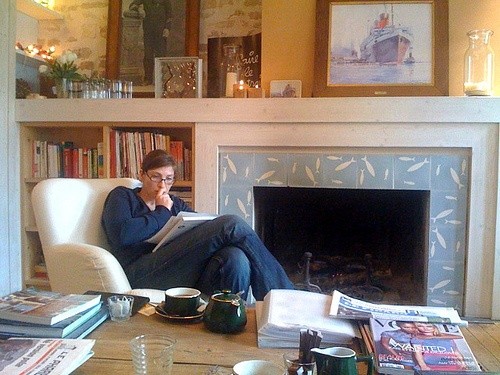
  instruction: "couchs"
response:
[31,178,144,295]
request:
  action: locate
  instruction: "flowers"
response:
[39,51,84,82]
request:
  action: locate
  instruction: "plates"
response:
[131,288,165,303]
[154,299,206,321]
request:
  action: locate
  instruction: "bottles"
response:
[218,44,243,98]
[462,29,495,96]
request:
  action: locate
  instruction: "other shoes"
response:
[140,80,153,86]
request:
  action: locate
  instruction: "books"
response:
[144,210,218,253]
[256,289,484,375]
[0,286,110,344]
[31,131,191,181]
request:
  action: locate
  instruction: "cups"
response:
[311,347,374,375]
[283,351,316,375]
[81,76,132,100]
[165,288,201,317]
[107,295,134,322]
[129,334,176,375]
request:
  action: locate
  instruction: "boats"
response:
[358,4,414,66]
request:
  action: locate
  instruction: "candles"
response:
[233,81,265,98]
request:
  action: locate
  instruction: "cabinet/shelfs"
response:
[17,123,195,295]
[8,0,64,99]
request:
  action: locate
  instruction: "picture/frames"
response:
[269,79,302,98]
[105,0,199,98]
[312,0,450,97]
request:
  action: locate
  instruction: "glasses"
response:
[145,171,175,184]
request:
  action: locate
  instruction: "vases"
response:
[55,79,69,99]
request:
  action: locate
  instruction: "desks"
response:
[63,299,500,375]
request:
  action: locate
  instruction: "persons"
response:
[100,149,294,305]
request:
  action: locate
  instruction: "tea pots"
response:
[204,289,247,332]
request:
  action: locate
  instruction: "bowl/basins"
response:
[232,360,286,375]
